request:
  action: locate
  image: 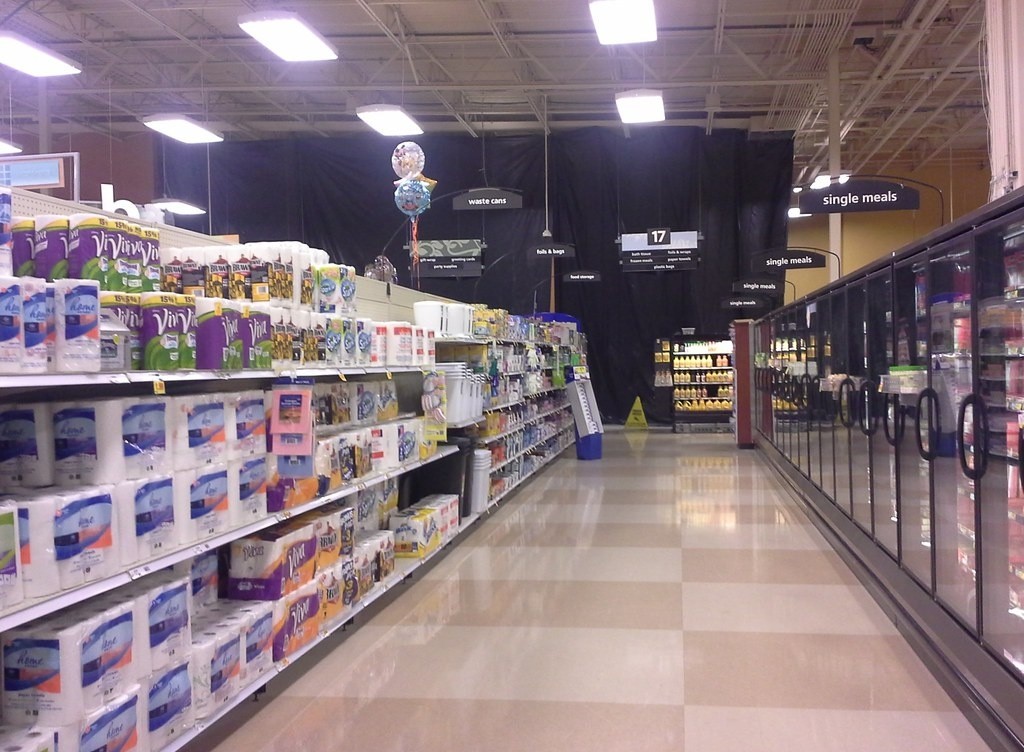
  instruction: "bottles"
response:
[717,370,727,382]
[686,386,696,398]
[673,371,680,383]
[692,400,705,412]
[696,371,701,382]
[706,371,717,381]
[714,399,729,411]
[691,356,701,368]
[701,371,705,382]
[702,355,712,367]
[674,356,679,367]
[680,371,690,382]
[697,386,707,397]
[718,386,729,397]
[655,371,672,386]
[716,355,728,367]
[706,399,714,411]
[680,355,691,368]
[675,400,691,412]
[674,386,685,398]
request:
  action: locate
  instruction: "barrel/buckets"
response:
[435,362,485,423]
[471,449,491,513]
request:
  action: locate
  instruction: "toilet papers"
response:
[0,194,587,752]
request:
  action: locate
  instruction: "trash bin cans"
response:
[435,362,485,423]
[472,449,492,513]
[413,301,476,339]
[409,436,475,526]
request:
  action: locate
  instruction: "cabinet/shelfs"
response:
[0,185,586,752]
[668,335,733,432]
